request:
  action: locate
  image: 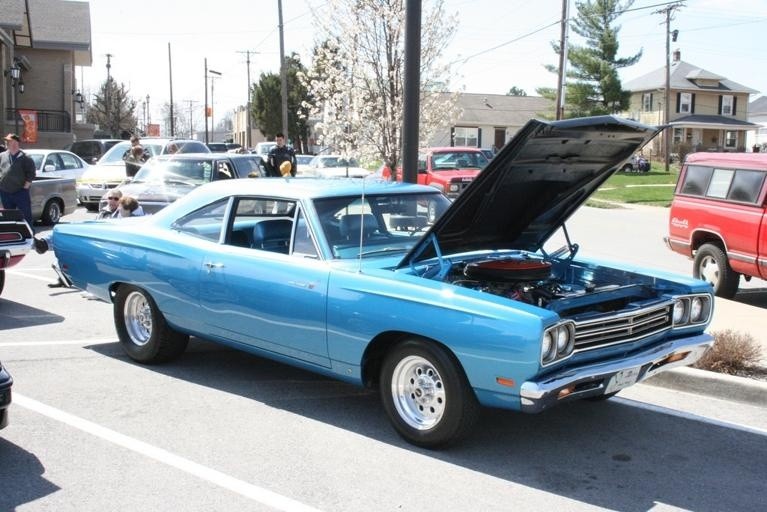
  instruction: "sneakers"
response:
[32,237,48,253]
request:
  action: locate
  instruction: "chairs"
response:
[42,160,54,171]
[253,219,293,250]
[456,160,467,168]
[340,214,377,240]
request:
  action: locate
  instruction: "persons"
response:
[33,187,122,251]
[0,133,37,233]
[116,196,141,218]
[267,133,298,177]
[276,162,295,215]
[123,137,180,176]
[247,169,266,213]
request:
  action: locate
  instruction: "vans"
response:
[379,145,489,203]
[662,151,766,300]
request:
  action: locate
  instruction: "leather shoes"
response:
[49,279,68,287]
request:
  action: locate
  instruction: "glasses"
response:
[108,197,119,201]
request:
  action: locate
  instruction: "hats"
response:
[4,134,20,141]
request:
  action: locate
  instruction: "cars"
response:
[50,116,715,449]
[619,152,639,173]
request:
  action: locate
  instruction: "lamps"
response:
[72,89,82,102]
[12,80,24,94]
[4,68,18,81]
[77,101,84,109]
